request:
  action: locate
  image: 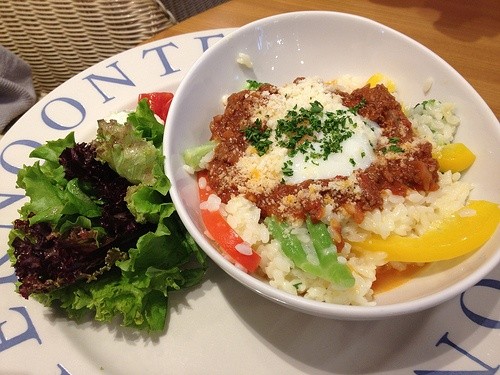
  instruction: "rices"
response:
[182,78,476,308]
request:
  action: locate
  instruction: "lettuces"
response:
[7,97,208,334]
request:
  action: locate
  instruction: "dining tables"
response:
[135,0,500,125]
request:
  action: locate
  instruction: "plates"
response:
[0,26,500,375]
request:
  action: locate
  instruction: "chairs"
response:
[0,0,179,102]
[160,0,230,23]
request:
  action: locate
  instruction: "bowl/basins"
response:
[163,9,500,321]
